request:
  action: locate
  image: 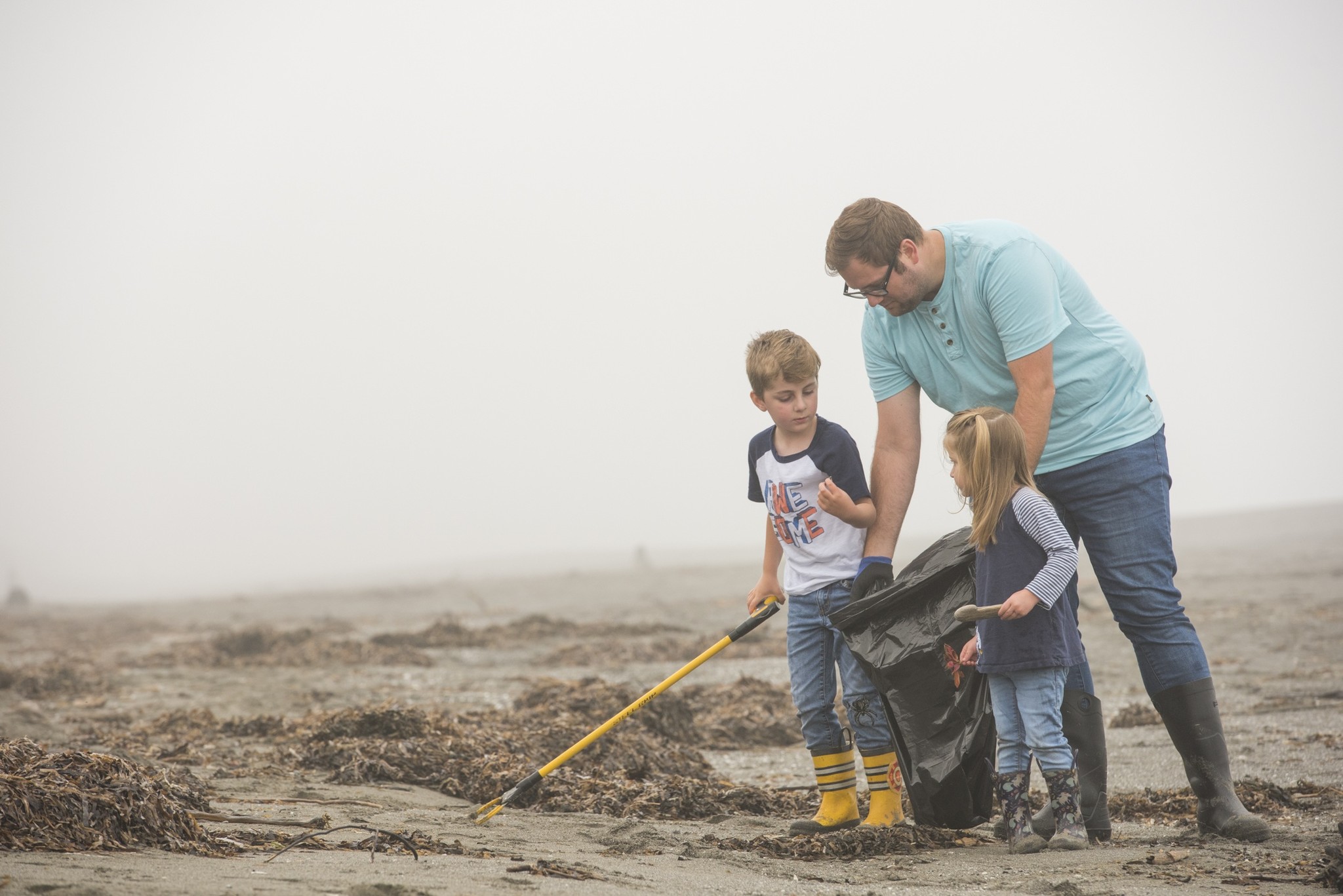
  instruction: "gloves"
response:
[849,557,895,606]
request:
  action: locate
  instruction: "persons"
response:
[746,329,905,832]
[937,403,1094,850]
[826,196,1274,839]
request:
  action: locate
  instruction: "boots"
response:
[789,727,861,837]
[1150,676,1272,843]
[993,689,1112,841]
[1047,748,1090,850]
[852,745,906,831]
[990,753,1047,854]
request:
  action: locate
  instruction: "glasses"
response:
[842,248,898,299]
